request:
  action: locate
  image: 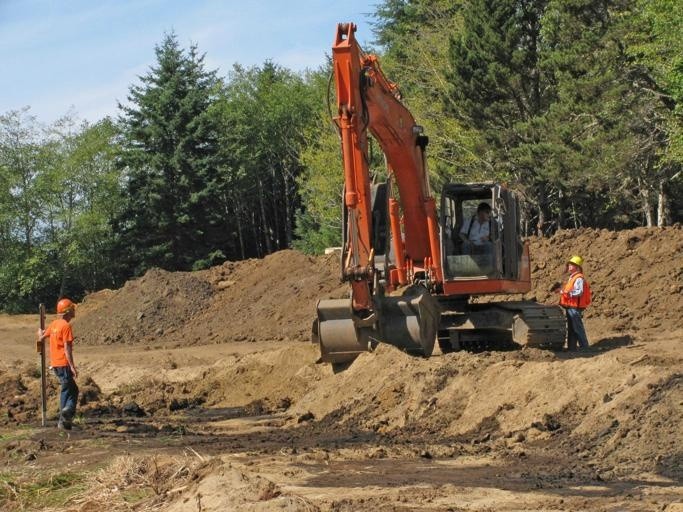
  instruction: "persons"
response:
[34,297,78,431]
[561,254,590,351]
[456,200,499,266]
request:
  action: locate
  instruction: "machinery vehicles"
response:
[303,17,570,371]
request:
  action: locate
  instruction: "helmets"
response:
[56,297,78,316]
[568,256,583,267]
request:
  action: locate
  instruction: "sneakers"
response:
[56,406,84,431]
